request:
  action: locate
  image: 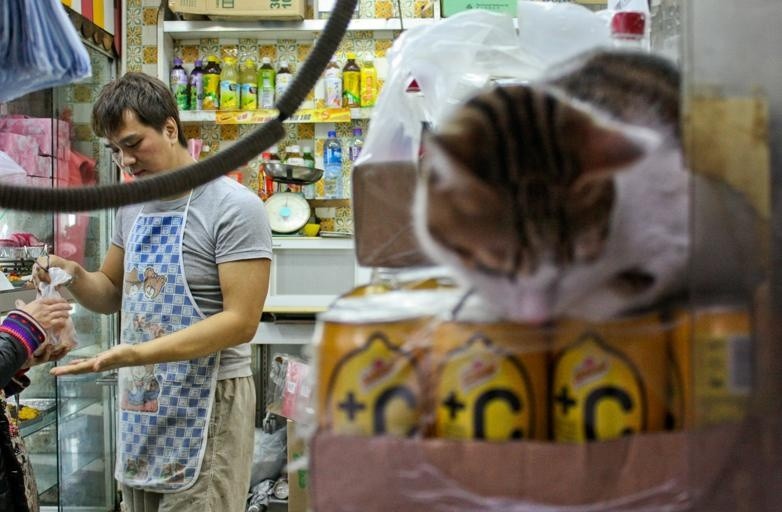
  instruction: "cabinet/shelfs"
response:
[155,1,443,313]
[0,0,124,512]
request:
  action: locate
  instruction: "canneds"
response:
[308,275,753,442]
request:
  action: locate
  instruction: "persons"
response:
[23,72,273,512]
[0,294,72,512]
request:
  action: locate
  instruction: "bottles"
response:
[195,127,365,199]
[299,52,378,110]
[188,58,204,110]
[257,56,275,110]
[241,58,257,111]
[219,53,241,111]
[274,60,293,105]
[204,56,221,110]
[170,57,189,111]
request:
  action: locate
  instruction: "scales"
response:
[262,163,324,236]
